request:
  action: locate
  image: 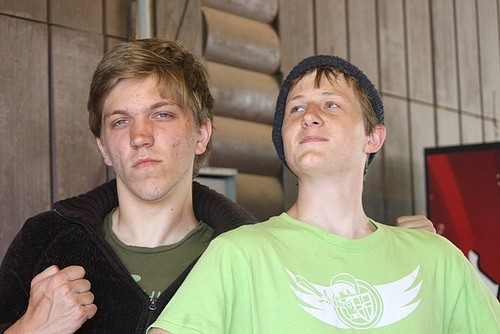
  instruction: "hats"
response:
[271,55,385,168]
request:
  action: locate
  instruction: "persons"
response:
[145,55,500,334]
[1,39,435,334]
